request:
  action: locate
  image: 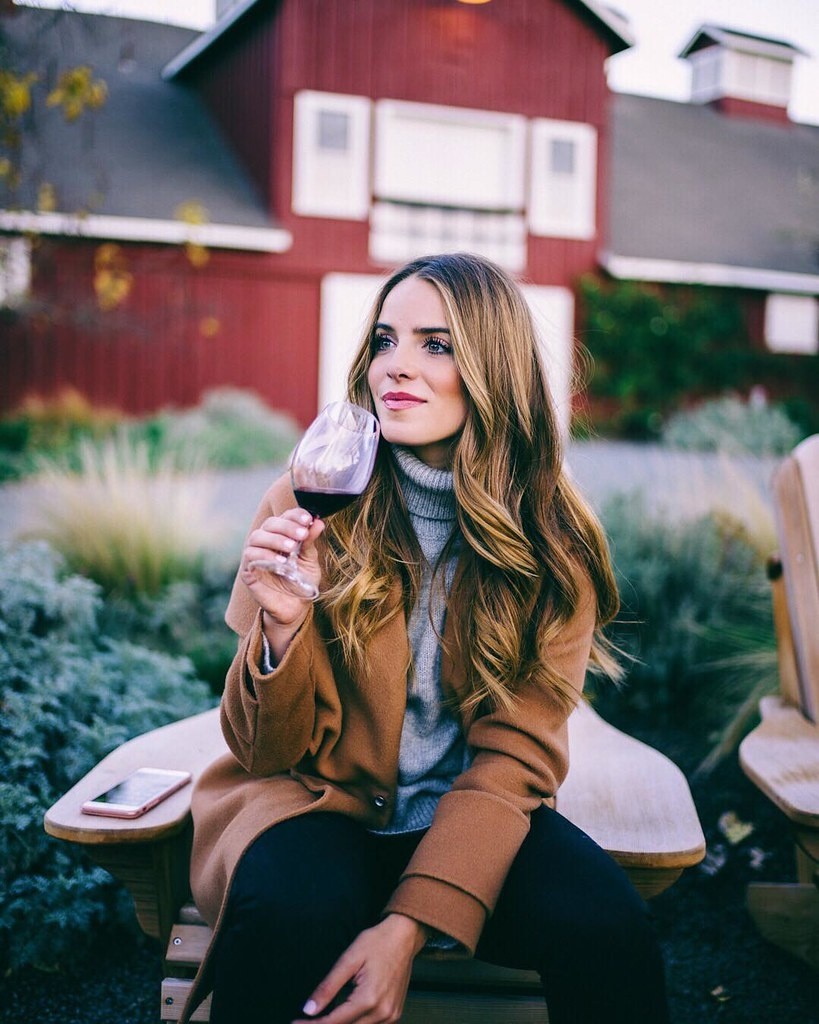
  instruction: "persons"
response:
[180,253,669,1024]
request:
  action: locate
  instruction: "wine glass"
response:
[248,401,379,600]
